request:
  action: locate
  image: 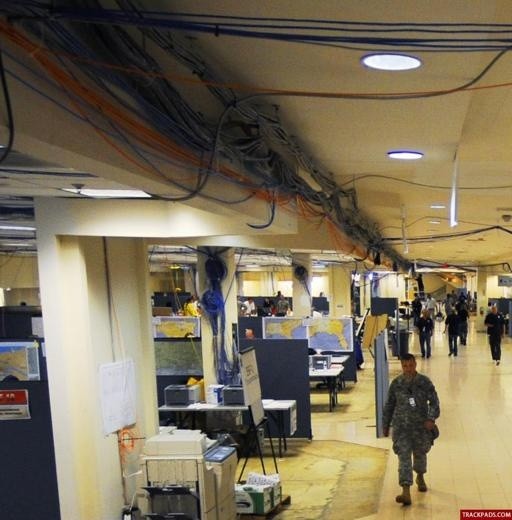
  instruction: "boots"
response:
[415,472,426,491]
[396,485,411,505]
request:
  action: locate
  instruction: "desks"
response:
[309,354,351,412]
[158,398,297,459]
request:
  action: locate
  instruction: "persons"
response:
[379,352,441,511]
[484,303,506,364]
[241,291,291,320]
[409,288,473,359]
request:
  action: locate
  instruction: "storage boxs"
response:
[235,481,282,514]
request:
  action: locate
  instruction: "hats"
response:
[428,424,439,445]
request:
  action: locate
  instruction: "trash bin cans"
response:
[390,330,412,356]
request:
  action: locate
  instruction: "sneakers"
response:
[449,351,457,357]
[492,359,500,366]
